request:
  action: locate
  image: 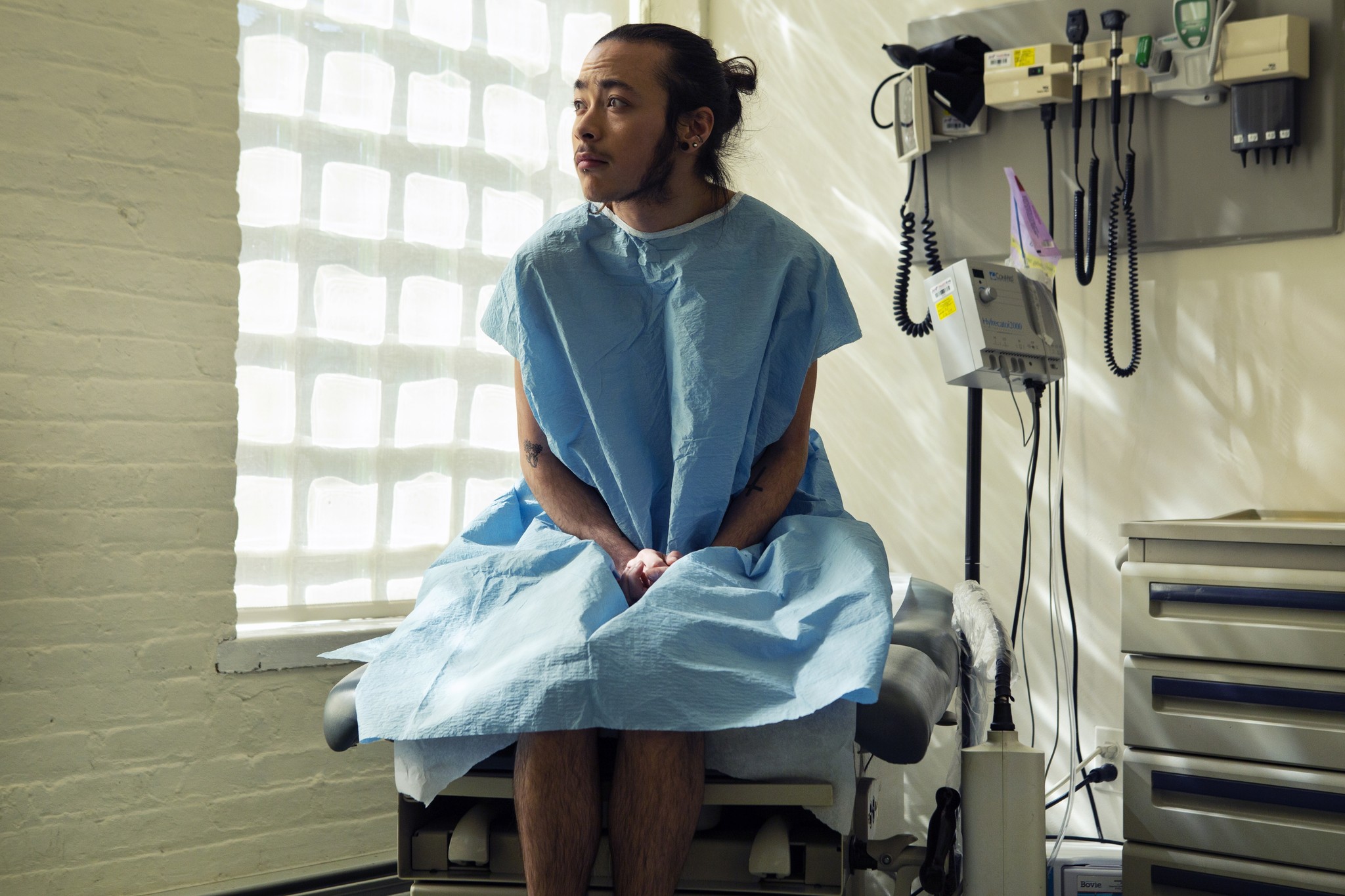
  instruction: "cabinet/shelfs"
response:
[1115,509,1345,896]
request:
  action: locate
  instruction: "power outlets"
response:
[1094,726,1124,793]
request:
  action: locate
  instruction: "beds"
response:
[320,577,963,896]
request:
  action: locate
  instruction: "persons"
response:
[479,23,863,896]
